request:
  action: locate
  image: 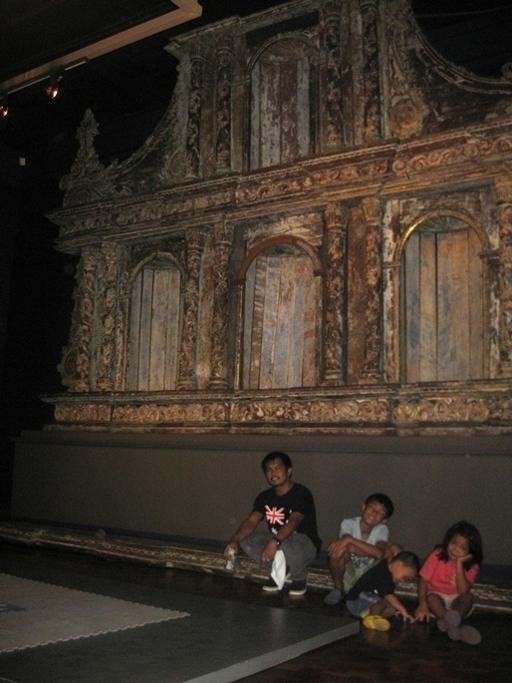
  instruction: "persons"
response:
[222,450,321,597]
[324,492,392,606]
[412,518,484,646]
[343,550,421,631]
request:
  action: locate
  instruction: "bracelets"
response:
[272,535,282,544]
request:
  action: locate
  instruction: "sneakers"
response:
[261,574,483,646]
